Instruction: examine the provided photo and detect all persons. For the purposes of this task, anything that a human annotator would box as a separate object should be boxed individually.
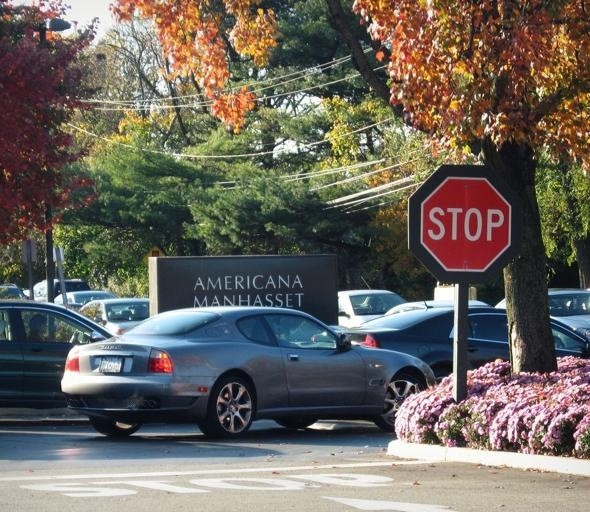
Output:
[26,313,48,342]
[369,297,385,313]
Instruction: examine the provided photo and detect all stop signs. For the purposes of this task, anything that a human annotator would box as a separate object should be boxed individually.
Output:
[419,176,512,273]
